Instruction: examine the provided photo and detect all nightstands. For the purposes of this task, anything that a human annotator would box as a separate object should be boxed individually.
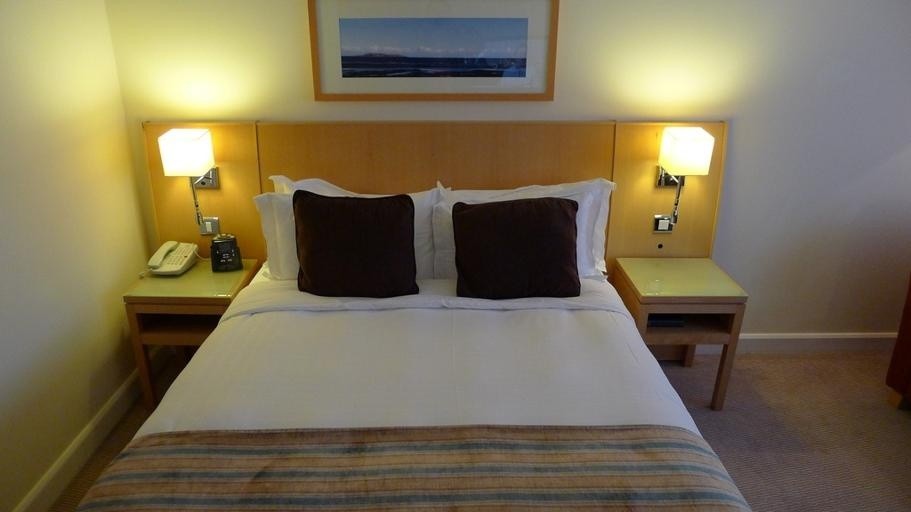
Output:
[123,258,261,411]
[613,259,749,412]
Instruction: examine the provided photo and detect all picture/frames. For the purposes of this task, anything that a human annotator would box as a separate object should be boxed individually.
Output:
[307,0,560,102]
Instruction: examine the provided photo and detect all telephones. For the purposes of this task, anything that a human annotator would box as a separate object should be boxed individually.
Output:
[147,241,198,275]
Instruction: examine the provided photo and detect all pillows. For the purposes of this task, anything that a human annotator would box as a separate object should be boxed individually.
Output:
[265,174,371,194]
[434,177,616,282]
[253,192,437,281]
[451,197,583,297]
[294,190,420,299]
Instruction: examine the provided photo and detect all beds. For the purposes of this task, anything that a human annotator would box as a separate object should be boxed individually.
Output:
[75,262,750,512]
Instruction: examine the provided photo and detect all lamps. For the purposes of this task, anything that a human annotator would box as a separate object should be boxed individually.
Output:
[656,126,716,226]
[156,128,220,226]
